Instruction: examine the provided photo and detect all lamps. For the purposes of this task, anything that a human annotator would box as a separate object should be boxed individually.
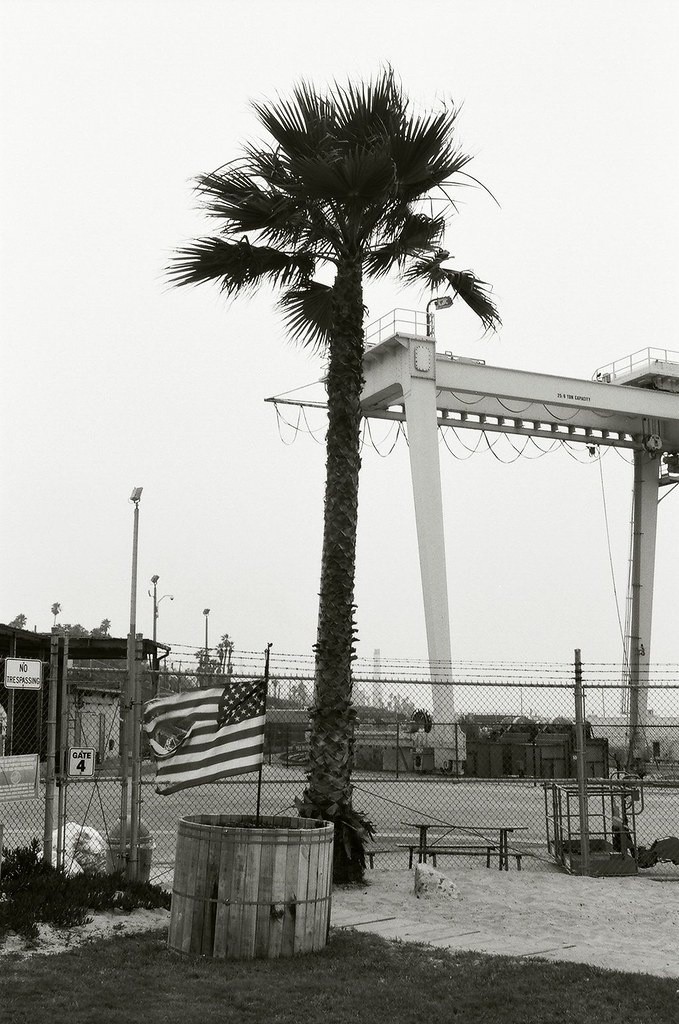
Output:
[427,296,454,337]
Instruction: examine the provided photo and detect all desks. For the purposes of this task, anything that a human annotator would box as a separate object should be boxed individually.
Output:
[400,821,529,871]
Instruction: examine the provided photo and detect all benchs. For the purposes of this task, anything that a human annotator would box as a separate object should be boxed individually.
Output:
[363,848,394,870]
[414,849,527,872]
[395,843,496,869]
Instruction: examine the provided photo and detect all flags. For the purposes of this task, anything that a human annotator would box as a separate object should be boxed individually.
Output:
[140,680,267,796]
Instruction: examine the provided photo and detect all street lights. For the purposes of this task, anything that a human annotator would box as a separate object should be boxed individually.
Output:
[201,609,210,685]
[125,486,146,883]
[152,593,174,674]
